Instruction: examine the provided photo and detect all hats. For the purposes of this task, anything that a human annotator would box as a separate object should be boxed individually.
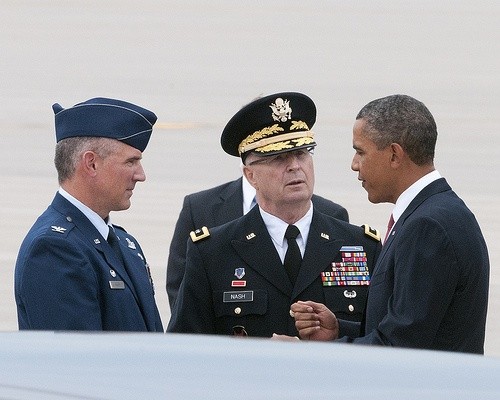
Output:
[221,92,316,167]
[52,98,157,153]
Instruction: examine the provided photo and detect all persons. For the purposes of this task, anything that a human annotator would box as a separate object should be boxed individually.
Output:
[14,97,165,333]
[271,94,490,355]
[165,90,384,342]
[166,175,349,314]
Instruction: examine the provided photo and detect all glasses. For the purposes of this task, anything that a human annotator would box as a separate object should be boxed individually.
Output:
[249,146,314,166]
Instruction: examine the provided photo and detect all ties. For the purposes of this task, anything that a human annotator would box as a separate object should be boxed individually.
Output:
[284,225,302,289]
[385,214,395,242]
[107,225,124,266]
